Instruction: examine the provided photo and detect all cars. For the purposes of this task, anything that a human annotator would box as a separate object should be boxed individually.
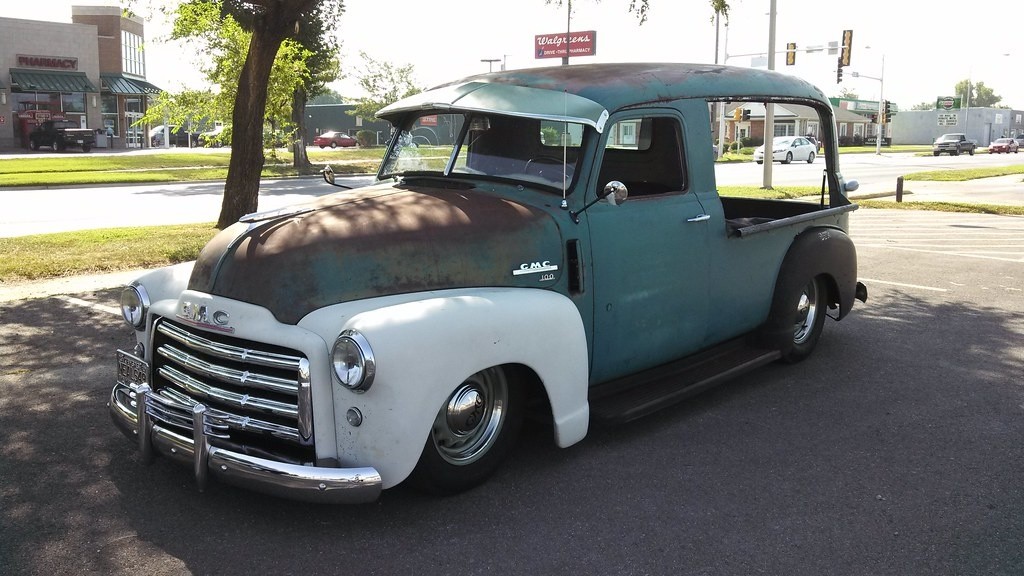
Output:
[313,132,358,149]
[1016,135,1024,146]
[126,128,144,142]
[987,137,1020,154]
[107,64,867,506]
[263,130,287,148]
[753,136,817,164]
[199,126,233,148]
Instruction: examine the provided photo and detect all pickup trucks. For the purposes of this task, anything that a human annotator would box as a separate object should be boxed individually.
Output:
[27,120,96,154]
[142,124,201,147]
[932,134,979,156]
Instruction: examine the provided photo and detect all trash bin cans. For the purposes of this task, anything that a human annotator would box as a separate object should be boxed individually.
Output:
[93,129,108,150]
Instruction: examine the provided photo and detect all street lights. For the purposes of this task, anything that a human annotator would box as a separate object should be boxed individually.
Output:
[481,59,501,72]
[964,52,1009,136]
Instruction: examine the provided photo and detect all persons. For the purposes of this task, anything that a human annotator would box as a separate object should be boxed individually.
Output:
[107,127,114,135]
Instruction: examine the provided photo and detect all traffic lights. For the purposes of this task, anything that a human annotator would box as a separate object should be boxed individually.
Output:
[884,101,891,124]
[837,59,844,83]
[743,110,751,122]
[734,108,741,121]
[873,115,878,123]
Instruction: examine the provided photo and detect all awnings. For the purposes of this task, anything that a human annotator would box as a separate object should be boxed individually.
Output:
[100,73,142,94]
[125,78,167,94]
[8,68,98,93]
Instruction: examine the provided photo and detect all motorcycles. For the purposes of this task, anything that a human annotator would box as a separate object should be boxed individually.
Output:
[386,131,419,149]
